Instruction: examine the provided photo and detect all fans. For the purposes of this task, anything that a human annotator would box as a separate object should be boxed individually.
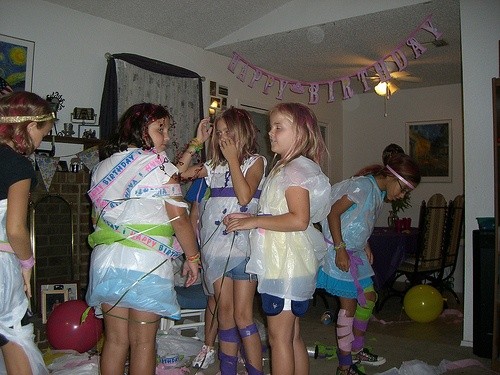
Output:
[352,69,421,84]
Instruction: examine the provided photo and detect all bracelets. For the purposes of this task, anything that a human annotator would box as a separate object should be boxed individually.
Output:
[187,136,203,153]
[333,241,346,252]
[184,250,201,263]
[18,256,36,269]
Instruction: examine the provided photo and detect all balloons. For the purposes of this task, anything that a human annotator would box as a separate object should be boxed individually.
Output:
[46,299,103,353]
[404,286,448,324]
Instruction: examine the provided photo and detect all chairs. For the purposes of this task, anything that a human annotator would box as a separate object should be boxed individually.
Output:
[380,194,447,316]
[159,284,206,340]
[426,194,465,304]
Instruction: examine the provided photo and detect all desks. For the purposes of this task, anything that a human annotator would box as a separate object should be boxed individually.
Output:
[367,226,419,313]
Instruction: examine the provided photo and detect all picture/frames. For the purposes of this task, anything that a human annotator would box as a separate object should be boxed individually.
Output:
[42,289,68,324]
[404,119,455,183]
[0,33,36,98]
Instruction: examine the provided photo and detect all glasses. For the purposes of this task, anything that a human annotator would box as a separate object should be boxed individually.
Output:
[397,179,406,193]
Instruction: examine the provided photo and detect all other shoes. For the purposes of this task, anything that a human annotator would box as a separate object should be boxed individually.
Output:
[336,364,366,375]
[352,348,377,362]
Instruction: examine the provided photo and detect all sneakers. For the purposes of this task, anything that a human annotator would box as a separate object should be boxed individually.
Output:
[192,344,216,369]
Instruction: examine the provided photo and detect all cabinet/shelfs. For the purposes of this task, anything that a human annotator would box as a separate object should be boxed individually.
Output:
[472,228,497,358]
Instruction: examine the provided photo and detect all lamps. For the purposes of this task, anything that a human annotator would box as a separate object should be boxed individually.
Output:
[373,81,402,97]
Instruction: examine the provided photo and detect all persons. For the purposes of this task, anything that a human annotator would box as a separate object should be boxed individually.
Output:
[86,104,200,375]
[223,102,331,375]
[0,91,58,375]
[174,108,268,375]
[314,153,422,375]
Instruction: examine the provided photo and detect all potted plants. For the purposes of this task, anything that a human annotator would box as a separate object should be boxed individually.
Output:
[387,191,411,229]
